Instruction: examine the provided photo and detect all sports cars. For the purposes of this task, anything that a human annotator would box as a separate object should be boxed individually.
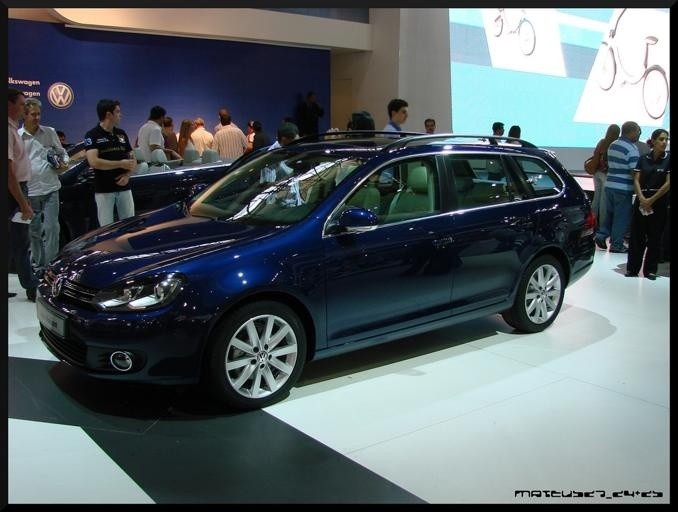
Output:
[7,139,233,290]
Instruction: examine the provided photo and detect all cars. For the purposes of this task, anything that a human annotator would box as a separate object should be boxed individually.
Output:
[29,130,595,408]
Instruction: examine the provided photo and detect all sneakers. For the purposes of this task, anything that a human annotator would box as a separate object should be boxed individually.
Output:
[593,234,608,250]
[609,243,629,254]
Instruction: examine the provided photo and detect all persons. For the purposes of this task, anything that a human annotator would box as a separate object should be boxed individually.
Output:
[8,88,302,303]
[380,99,409,181]
[350,111,375,138]
[591,121,670,280]
[506,125,520,145]
[297,91,325,137]
[425,118,435,134]
[485,123,504,181]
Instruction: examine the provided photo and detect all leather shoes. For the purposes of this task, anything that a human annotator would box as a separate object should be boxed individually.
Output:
[625,270,638,277]
[644,272,657,280]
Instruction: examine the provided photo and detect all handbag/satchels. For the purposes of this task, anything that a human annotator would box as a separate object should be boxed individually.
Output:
[584,154,600,176]
